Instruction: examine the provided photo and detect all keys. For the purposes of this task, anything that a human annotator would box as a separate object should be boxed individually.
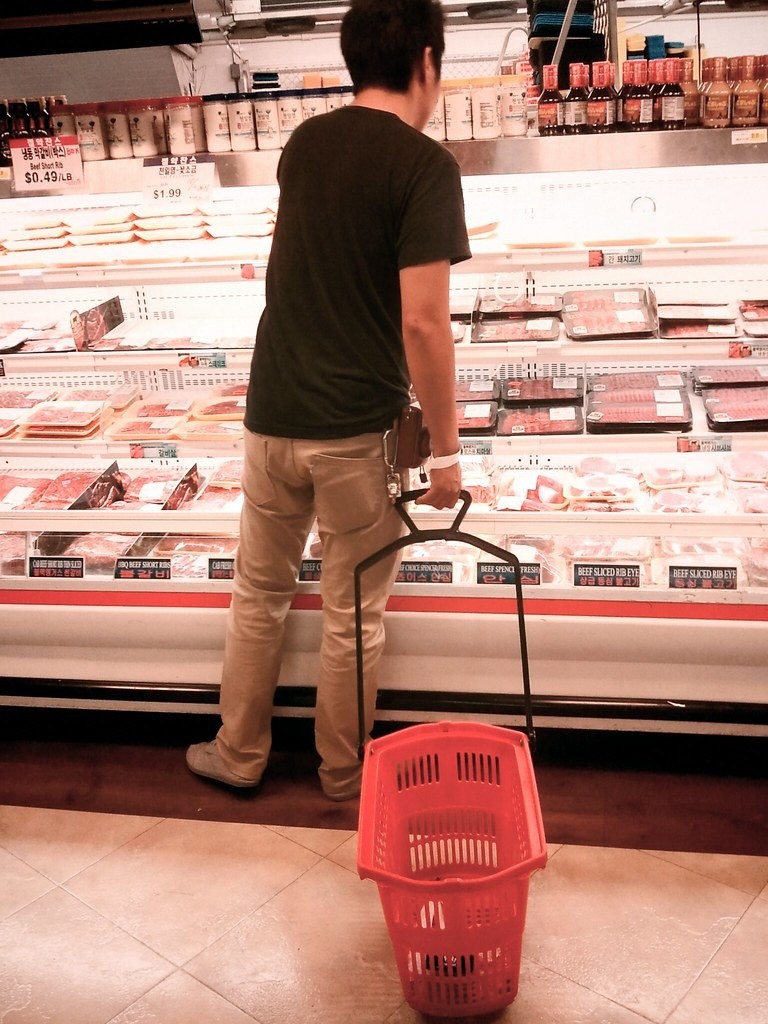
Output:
[385,474,401,505]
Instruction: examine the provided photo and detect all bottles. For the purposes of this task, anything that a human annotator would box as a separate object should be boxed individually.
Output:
[420,56,766,140]
[0,85,355,167]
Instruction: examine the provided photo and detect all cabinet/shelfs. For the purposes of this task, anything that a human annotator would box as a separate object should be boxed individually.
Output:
[0,30,767,731]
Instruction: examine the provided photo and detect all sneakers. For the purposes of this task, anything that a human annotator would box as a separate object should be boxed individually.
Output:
[185,741,262,797]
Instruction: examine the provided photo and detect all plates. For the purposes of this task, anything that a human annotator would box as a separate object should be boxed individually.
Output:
[1,192,280,253]
[0,284,767,588]
[466,212,767,247]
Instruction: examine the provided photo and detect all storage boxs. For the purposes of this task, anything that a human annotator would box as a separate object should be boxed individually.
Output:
[355,715,548,1024]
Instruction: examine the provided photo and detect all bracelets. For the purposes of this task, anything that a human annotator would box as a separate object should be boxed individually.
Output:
[429,443,464,470]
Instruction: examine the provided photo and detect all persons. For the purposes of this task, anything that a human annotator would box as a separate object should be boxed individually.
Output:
[187,0,474,802]
